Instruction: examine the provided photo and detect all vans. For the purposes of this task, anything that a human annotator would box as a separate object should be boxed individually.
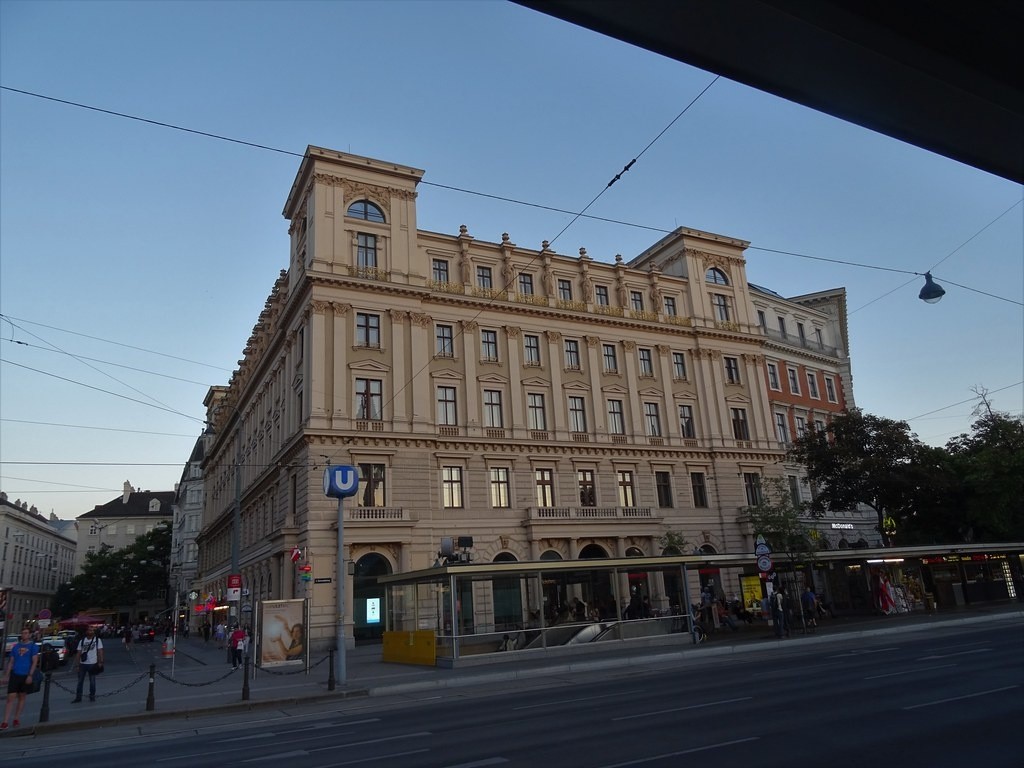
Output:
[58,630,76,640]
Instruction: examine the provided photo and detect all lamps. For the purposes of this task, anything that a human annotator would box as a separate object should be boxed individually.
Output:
[919,274,945,305]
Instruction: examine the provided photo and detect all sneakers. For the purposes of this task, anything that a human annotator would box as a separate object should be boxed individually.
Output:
[13,720,21,727]
[0,723,9,729]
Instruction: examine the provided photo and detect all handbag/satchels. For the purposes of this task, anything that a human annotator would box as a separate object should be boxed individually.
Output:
[23,680,41,694]
[91,663,105,674]
[121,637,126,643]
[80,652,87,662]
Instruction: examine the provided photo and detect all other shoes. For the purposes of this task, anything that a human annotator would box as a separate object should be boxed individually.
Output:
[239,664,243,670]
[90,697,95,702]
[72,697,82,703]
[231,666,238,670]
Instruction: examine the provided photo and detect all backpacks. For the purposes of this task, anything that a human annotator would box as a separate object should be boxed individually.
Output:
[770,592,779,611]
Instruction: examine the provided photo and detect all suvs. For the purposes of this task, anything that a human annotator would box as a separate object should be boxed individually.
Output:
[140,628,154,641]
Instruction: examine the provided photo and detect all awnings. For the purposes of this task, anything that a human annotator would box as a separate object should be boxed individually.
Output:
[159,606,175,616]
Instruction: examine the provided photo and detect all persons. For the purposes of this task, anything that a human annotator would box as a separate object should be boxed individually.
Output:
[770,586,794,639]
[499,628,526,651]
[599,595,617,622]
[574,597,586,622]
[695,587,726,626]
[71,628,104,703]
[214,620,252,669]
[458,253,664,309]
[0,628,40,729]
[525,611,549,637]
[198,621,211,646]
[147,621,190,639]
[802,586,819,627]
[272,614,304,660]
[552,605,560,625]
[97,624,143,650]
[621,583,655,620]
[749,592,761,611]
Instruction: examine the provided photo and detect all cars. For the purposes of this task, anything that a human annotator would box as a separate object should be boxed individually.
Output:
[5,637,20,653]
[43,640,69,665]
[35,643,59,672]
